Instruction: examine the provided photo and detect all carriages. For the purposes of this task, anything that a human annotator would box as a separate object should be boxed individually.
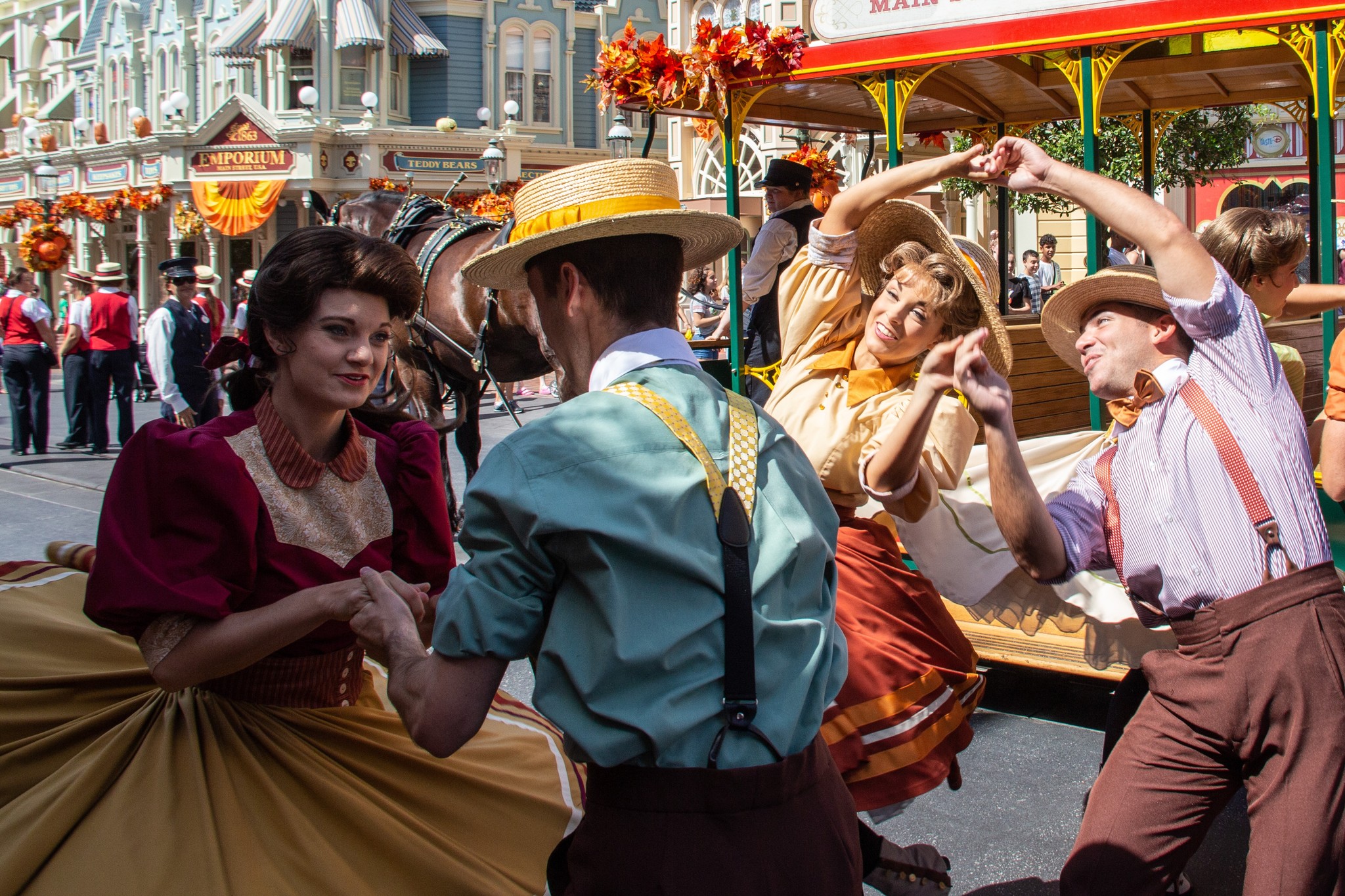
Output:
[309,0,1345,680]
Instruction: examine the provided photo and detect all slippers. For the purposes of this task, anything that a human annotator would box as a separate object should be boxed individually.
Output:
[516,387,534,396]
[538,386,552,394]
[443,405,454,411]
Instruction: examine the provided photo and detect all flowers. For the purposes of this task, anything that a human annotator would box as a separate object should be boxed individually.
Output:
[367,177,527,224]
[583,17,811,119]
[0,181,206,272]
[782,144,842,189]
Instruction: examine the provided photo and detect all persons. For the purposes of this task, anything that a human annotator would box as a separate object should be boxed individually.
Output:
[954,135,1345,896]
[441,383,453,410]
[0,267,59,456]
[368,341,395,410]
[516,375,551,396]
[232,269,259,368]
[493,382,524,414]
[762,143,1012,812]
[676,257,754,362]
[703,159,825,407]
[144,257,226,429]
[1196,207,1345,502]
[78,226,588,896]
[351,158,868,896]
[55,262,151,455]
[1108,229,1144,267]
[989,229,1066,315]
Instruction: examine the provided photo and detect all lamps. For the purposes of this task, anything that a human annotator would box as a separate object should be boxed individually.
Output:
[298,86,319,112]
[477,107,492,126]
[504,100,520,120]
[73,117,90,136]
[361,92,379,114]
[160,91,190,122]
[24,126,39,145]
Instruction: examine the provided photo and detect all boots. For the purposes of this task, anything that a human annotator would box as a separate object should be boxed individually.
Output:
[863,835,953,896]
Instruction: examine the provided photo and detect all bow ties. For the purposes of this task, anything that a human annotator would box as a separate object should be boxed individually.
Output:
[1104,368,1166,428]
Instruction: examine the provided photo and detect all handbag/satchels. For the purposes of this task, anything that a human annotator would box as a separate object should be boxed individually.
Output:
[1041,293,1055,304]
[684,328,693,340]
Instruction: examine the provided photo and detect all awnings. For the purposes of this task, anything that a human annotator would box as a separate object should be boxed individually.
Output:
[0,94,16,129]
[46,11,80,42]
[389,0,450,60]
[334,0,385,53]
[208,0,314,69]
[0,29,15,58]
[35,86,74,121]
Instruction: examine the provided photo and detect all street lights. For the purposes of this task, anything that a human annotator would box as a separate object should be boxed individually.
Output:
[34,154,60,342]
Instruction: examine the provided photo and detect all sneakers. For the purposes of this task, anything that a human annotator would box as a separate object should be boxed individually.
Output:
[56,441,94,449]
[494,399,524,414]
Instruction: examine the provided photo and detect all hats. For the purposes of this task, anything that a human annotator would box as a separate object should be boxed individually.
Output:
[92,262,129,281]
[59,290,67,296]
[1040,263,1172,376]
[60,267,98,285]
[194,265,222,288]
[236,269,258,288]
[752,158,814,195]
[857,198,1014,384]
[462,159,747,292]
[158,257,199,278]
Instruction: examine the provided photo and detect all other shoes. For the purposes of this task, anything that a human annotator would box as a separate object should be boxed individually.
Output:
[446,389,455,395]
[441,395,454,404]
[93,444,109,454]
[444,394,453,399]
[11,448,24,456]
[0,389,8,394]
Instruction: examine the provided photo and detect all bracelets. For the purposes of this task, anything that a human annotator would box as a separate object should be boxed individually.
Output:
[685,324,690,326]
[53,352,58,354]
[718,315,721,320]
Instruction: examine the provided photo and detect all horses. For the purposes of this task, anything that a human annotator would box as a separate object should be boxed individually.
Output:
[308,189,577,541]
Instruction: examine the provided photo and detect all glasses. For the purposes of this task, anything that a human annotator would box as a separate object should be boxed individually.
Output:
[167,275,196,286]
